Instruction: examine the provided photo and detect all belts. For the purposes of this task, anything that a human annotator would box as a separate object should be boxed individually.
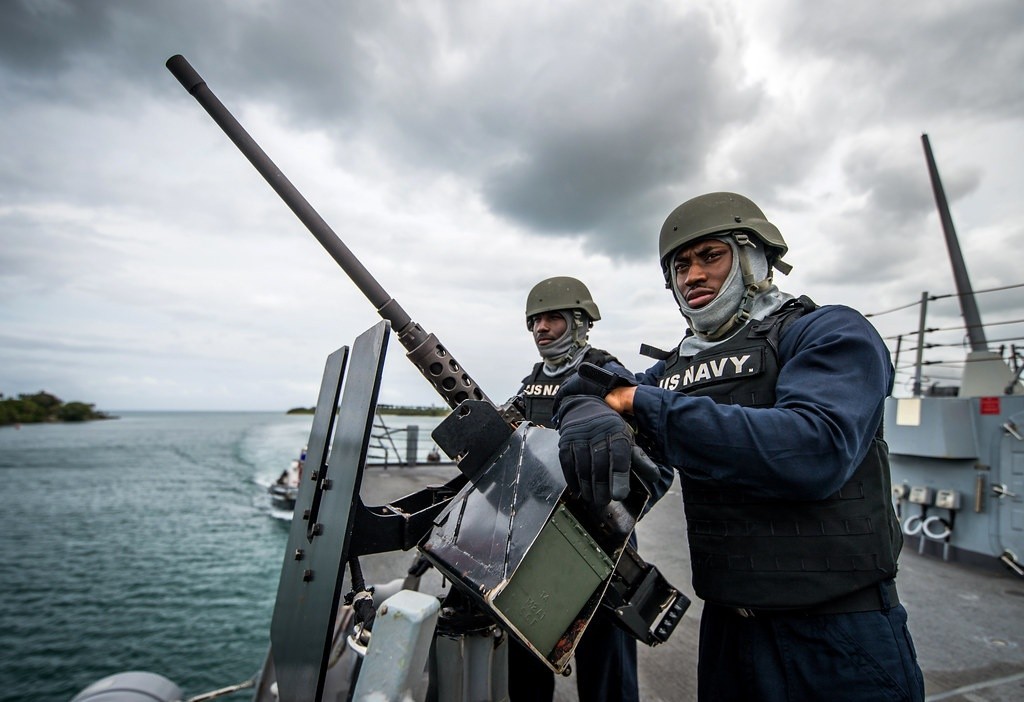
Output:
[732,583,900,620]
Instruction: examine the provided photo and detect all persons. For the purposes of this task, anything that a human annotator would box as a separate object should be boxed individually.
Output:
[507,277,674,702]
[551,191,927,702]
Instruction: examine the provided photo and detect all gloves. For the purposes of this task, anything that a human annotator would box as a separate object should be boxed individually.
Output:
[550,391,634,507]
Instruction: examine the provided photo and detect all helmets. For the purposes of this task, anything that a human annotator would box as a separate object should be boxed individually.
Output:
[659,191,788,261]
[525,276,601,320]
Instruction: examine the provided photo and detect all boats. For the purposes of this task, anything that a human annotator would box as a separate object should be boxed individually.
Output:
[269,461,299,512]
